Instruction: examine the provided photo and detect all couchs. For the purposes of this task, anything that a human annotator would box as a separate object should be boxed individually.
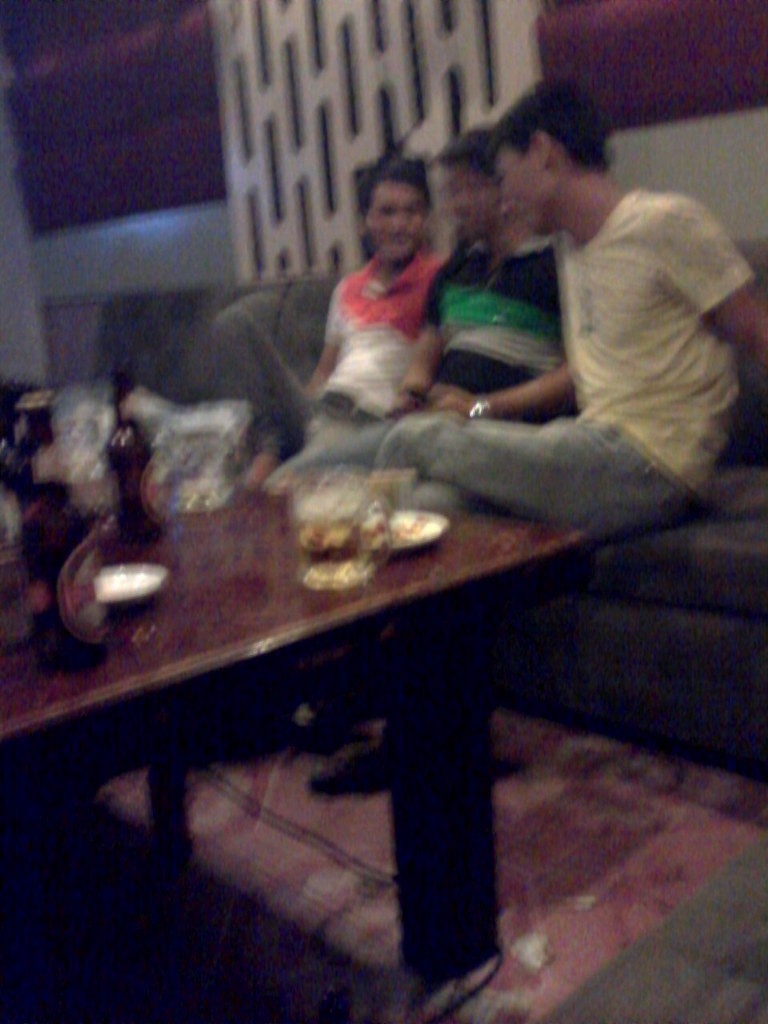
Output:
[198,236,768,783]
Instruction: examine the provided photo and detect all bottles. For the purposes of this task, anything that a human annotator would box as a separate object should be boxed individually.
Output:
[104,387,168,542]
[15,387,109,679]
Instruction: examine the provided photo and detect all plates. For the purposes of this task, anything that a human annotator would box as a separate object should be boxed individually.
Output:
[382,509,450,550]
[95,564,169,604]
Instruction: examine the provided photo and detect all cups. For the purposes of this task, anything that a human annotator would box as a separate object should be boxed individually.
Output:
[366,468,414,510]
[294,470,372,589]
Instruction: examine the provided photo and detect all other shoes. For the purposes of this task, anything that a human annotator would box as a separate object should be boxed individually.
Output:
[308,741,391,796]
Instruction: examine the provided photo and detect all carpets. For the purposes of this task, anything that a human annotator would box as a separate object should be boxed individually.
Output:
[95,703,768,1024]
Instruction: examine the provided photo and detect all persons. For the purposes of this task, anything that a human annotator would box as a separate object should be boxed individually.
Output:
[242,77,768,790]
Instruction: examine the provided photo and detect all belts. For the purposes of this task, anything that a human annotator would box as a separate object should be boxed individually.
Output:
[321,393,376,424]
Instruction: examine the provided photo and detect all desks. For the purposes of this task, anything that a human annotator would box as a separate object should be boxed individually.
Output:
[0,485,585,985]
